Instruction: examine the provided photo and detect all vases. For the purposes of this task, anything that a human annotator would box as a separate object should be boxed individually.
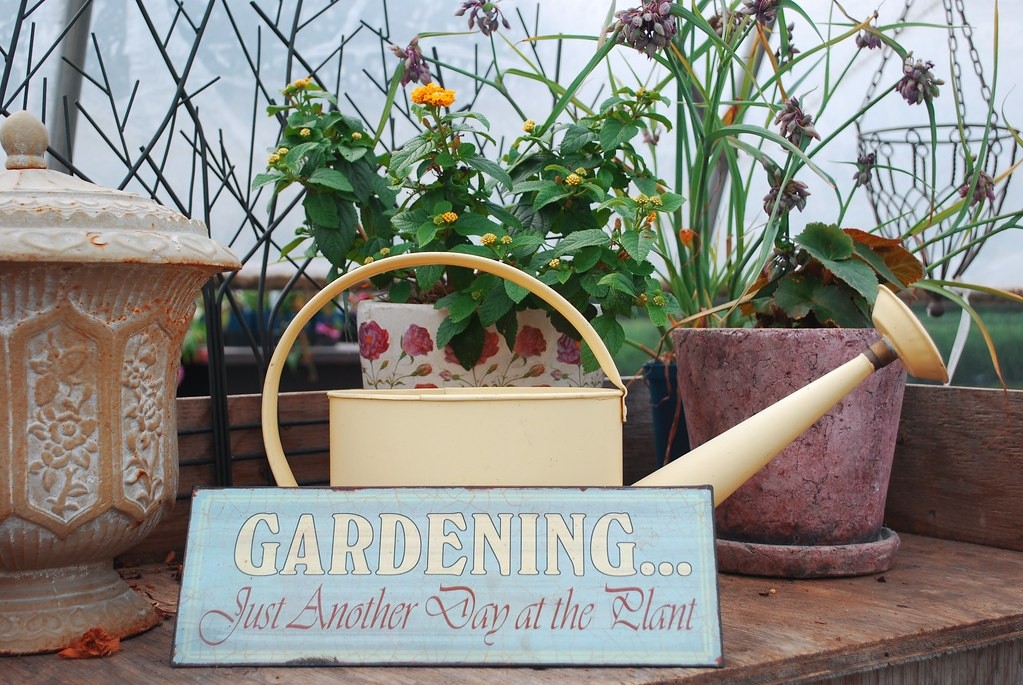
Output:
[668,323,911,576]
[354,294,611,389]
[327,384,626,487]
[643,359,690,468]
[241,0,1023,332]
